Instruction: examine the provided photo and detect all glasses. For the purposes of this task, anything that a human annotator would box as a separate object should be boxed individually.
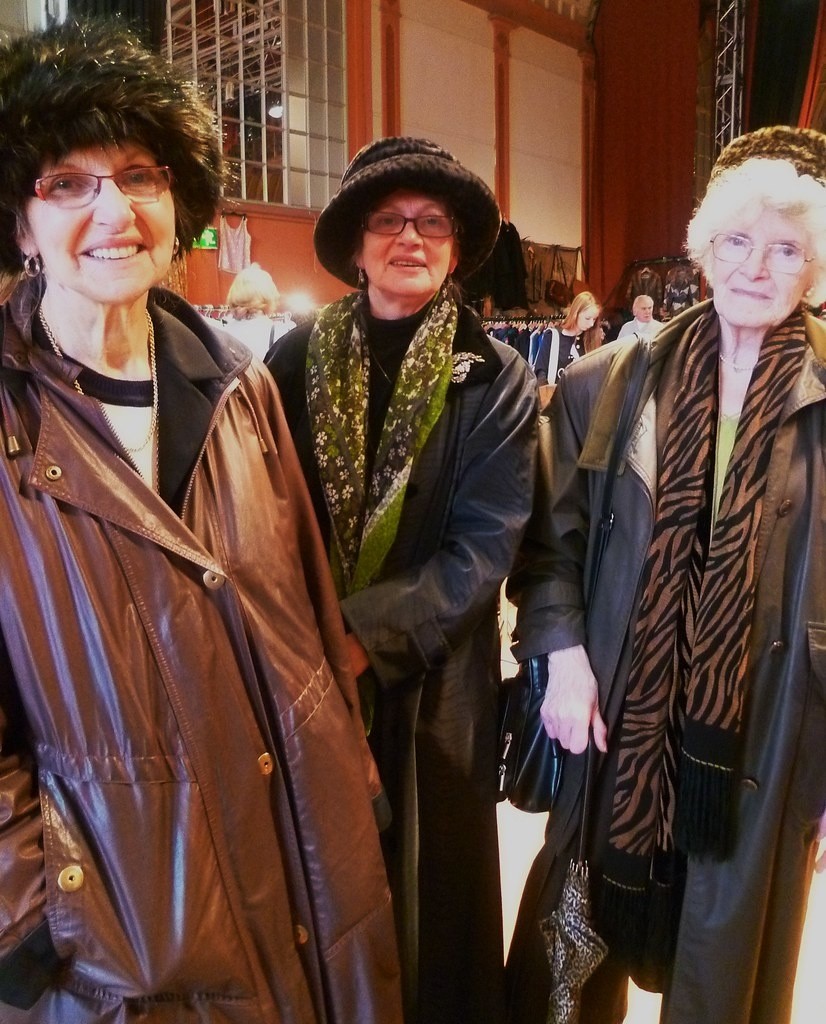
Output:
[361,210,464,239]
[29,165,174,210]
[709,232,816,274]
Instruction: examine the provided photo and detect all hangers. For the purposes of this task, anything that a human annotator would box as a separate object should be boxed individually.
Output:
[641,261,651,274]
[221,200,246,220]
[482,315,566,334]
[193,304,292,323]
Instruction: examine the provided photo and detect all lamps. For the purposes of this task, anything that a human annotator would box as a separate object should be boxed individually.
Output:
[225,51,234,102]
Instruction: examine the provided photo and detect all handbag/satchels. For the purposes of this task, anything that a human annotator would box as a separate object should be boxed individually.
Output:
[496,656,566,813]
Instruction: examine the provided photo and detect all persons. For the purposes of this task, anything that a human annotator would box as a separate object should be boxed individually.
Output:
[616,294,664,342]
[537,291,599,386]
[218,264,288,360]
[503,126,826,1024]
[261,138,543,1024]
[0,16,401,1024]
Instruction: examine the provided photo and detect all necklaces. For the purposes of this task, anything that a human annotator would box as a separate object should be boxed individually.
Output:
[37,305,157,449]
[369,350,393,386]
[719,357,755,373]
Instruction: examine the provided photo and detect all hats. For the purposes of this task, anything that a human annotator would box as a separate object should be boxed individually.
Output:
[0,13,245,271]
[314,136,503,289]
